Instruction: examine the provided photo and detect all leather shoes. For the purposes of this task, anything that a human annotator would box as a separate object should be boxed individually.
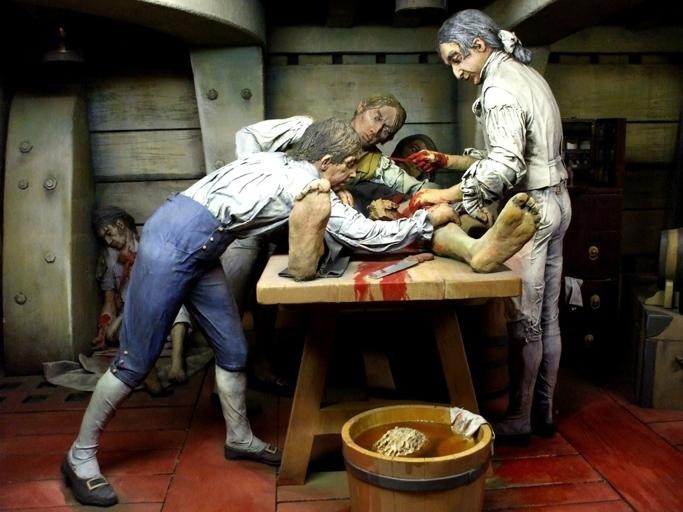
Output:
[60,454,119,506]
[224,444,282,465]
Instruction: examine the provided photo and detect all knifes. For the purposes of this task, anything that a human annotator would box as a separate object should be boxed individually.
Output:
[375,253,433,280]
[386,156,413,164]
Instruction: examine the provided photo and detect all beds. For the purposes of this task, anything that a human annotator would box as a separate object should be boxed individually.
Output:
[252,244,533,486]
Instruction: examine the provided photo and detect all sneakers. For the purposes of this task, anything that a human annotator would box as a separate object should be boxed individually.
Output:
[494,423,556,449]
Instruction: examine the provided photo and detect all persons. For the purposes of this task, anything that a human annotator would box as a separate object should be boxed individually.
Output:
[207,91,447,414]
[285,130,543,285]
[89,201,193,393]
[402,7,575,453]
[56,115,363,508]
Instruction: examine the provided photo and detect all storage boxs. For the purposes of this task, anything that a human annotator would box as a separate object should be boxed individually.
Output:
[637,301,682,411]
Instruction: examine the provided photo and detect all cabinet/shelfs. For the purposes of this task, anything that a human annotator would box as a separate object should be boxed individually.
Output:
[558,112,629,394]
